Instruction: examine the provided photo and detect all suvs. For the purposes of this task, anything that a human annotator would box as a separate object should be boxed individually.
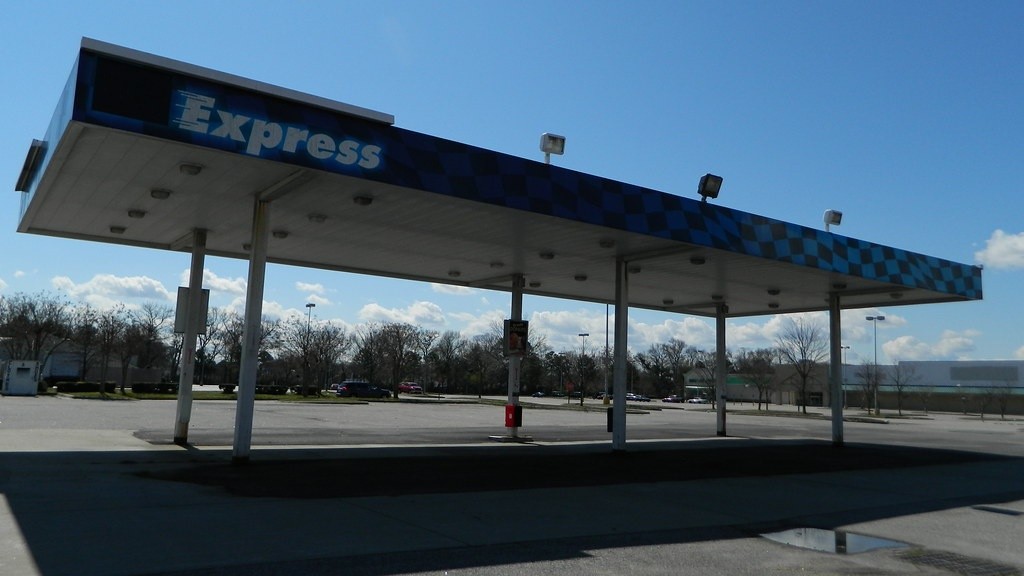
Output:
[336,380,392,399]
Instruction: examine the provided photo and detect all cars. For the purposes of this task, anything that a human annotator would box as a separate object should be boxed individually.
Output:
[597,391,614,400]
[399,380,424,394]
[662,395,683,403]
[330,384,339,389]
[626,392,651,402]
[552,390,564,397]
[572,391,582,400]
[687,397,709,405]
[532,391,545,397]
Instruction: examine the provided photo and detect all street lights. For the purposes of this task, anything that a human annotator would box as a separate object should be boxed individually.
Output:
[305,302,316,351]
[840,345,851,409]
[578,332,589,406]
[866,314,885,416]
